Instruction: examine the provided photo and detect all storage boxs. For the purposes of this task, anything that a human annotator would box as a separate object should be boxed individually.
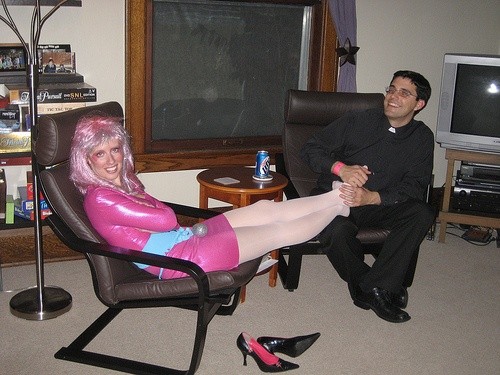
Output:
[9,81,97,104]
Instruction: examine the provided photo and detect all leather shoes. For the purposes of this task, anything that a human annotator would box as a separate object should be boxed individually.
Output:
[354,285,412,322]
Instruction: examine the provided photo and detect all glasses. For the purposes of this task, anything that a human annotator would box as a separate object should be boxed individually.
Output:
[384,86,418,99]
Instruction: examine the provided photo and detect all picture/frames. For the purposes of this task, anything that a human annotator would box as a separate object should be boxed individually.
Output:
[0,42,29,77]
[37,44,71,73]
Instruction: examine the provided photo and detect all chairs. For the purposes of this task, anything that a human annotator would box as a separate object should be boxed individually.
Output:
[276,89,435,292]
[35,101,262,375]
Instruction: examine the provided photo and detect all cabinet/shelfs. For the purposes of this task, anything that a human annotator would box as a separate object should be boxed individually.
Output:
[0,101,96,267]
[438,149,500,244]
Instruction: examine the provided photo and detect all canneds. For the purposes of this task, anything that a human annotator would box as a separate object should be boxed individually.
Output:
[256,150,270,178]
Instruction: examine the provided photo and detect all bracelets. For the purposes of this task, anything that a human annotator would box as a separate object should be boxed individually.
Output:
[334,162,345,176]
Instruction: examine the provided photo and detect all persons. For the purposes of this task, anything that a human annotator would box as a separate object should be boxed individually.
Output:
[303,70,435,323]
[69,116,368,279]
[0,54,22,69]
[44,59,72,73]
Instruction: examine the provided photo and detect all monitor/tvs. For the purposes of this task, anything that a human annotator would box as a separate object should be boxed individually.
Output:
[435,53,500,155]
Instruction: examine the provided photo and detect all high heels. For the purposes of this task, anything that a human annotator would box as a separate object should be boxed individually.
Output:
[236,332,300,373]
[257,332,321,358]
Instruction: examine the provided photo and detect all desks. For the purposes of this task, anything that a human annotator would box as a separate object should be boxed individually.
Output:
[196,167,289,301]
